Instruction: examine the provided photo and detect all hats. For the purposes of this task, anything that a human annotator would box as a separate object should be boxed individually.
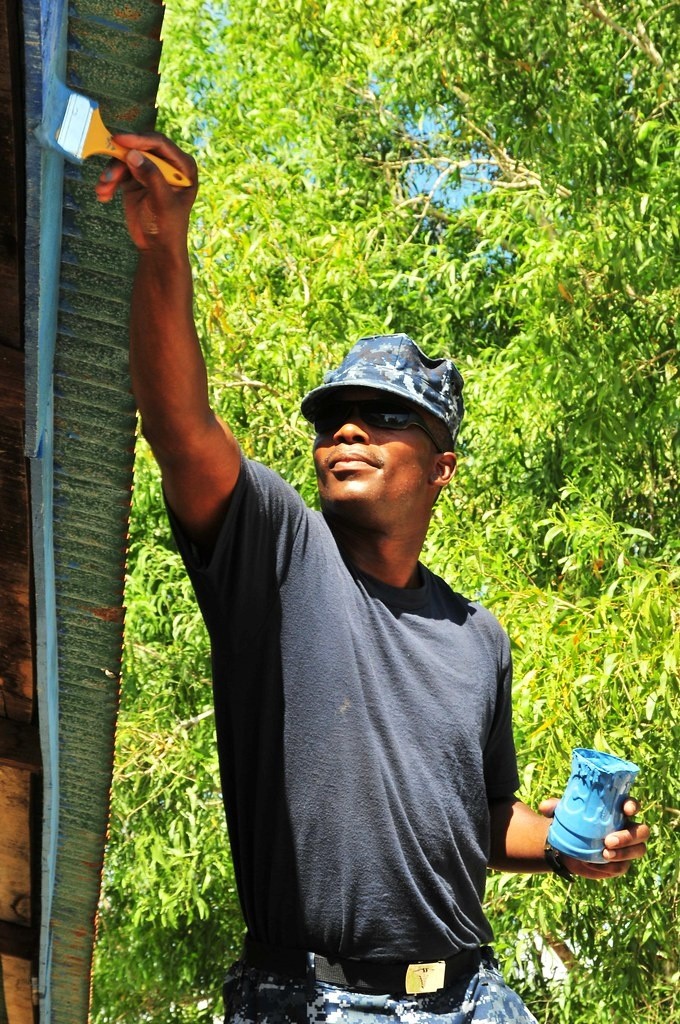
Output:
[302,332,463,443]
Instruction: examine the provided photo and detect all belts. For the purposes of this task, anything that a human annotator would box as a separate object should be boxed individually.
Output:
[243,933,480,997]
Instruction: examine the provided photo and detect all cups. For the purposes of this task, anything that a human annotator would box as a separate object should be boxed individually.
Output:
[546,747,639,865]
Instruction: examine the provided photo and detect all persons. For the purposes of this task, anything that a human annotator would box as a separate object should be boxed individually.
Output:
[98,127,650,1024]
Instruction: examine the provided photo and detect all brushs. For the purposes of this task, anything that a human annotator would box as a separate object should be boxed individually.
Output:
[33,71,192,187]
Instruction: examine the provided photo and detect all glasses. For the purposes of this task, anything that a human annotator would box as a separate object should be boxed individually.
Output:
[314,397,443,454]
